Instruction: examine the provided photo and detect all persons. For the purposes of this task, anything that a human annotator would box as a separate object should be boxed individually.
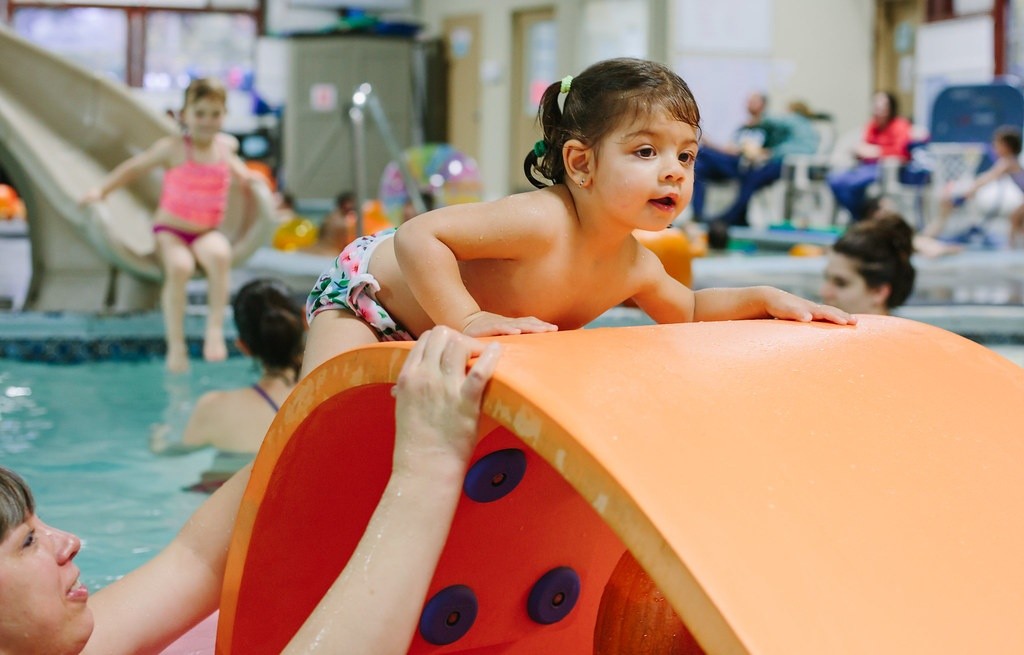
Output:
[301,58,858,386]
[83,83,1024,448]
[0,324,501,654]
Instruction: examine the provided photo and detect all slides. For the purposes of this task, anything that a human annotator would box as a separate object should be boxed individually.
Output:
[0,26,278,284]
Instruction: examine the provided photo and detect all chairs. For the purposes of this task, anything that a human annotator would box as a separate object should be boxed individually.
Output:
[746,115,837,224]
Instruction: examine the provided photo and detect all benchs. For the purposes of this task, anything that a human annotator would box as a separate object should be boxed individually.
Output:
[856,140,987,199]
